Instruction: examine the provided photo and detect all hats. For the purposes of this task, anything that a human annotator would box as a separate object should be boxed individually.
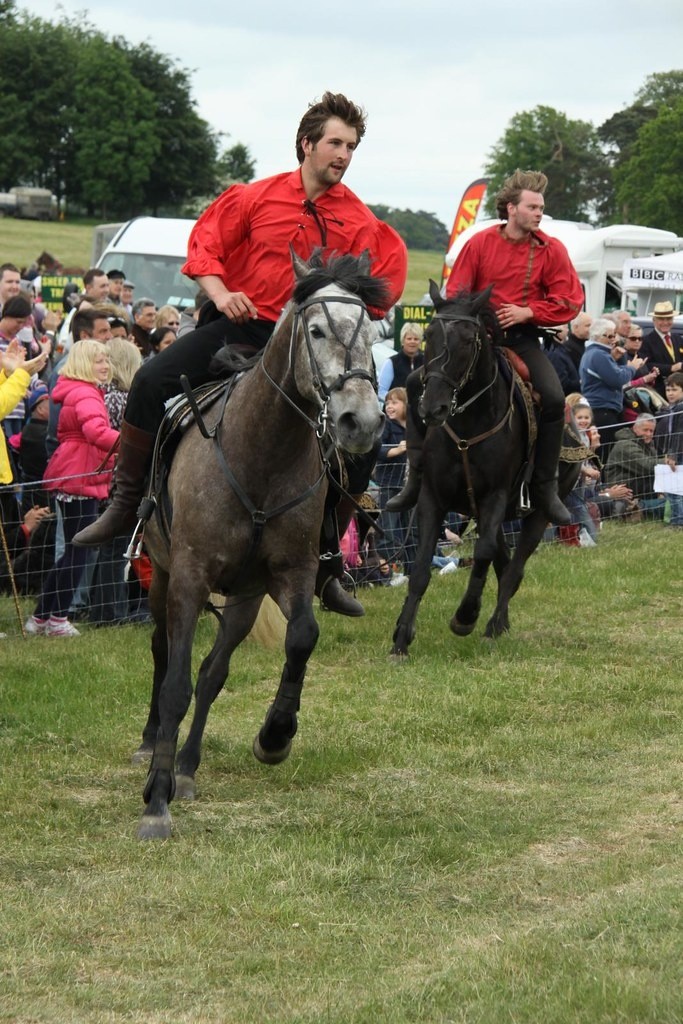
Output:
[123,279,135,289]
[648,301,679,318]
[28,386,50,410]
[107,270,125,280]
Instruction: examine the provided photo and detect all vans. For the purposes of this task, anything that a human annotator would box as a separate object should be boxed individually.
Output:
[46,215,203,369]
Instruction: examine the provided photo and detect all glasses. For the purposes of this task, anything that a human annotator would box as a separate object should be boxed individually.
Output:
[626,336,643,341]
[603,335,615,339]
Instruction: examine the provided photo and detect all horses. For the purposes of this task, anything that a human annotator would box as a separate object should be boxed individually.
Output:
[133,239,388,842]
[388,277,559,660]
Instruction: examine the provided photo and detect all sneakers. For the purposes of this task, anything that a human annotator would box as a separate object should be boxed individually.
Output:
[23,614,81,636]
[458,557,474,568]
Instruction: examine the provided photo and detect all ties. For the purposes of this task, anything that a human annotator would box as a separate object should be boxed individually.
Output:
[664,335,672,348]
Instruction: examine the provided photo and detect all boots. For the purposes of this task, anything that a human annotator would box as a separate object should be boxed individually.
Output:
[384,446,424,512]
[71,416,157,547]
[314,491,367,618]
[531,416,572,527]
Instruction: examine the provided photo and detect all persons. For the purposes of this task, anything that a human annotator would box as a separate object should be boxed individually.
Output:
[1,265,682,639]
[72,92,408,618]
[384,168,585,525]
